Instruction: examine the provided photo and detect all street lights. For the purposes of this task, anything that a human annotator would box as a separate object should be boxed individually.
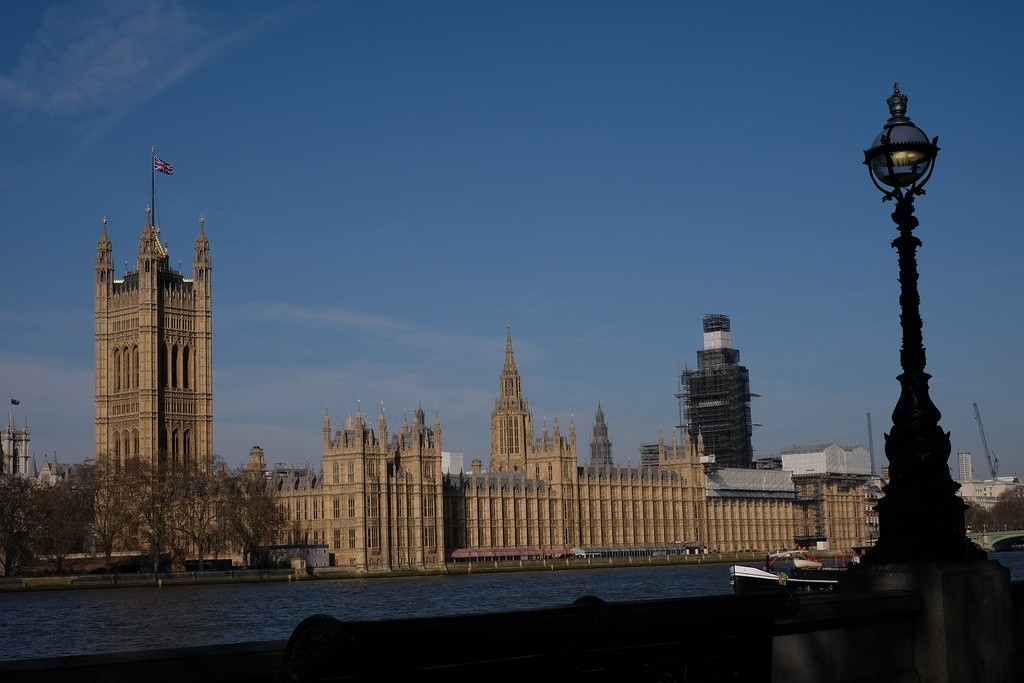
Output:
[856,85,991,567]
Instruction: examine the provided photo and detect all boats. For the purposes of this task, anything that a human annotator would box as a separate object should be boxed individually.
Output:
[727,548,851,592]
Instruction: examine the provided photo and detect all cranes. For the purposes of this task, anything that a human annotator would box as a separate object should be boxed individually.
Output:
[972,401,999,482]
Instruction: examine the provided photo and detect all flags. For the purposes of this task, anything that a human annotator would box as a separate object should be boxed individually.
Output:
[11,398,20,405]
[154,154,174,176]
